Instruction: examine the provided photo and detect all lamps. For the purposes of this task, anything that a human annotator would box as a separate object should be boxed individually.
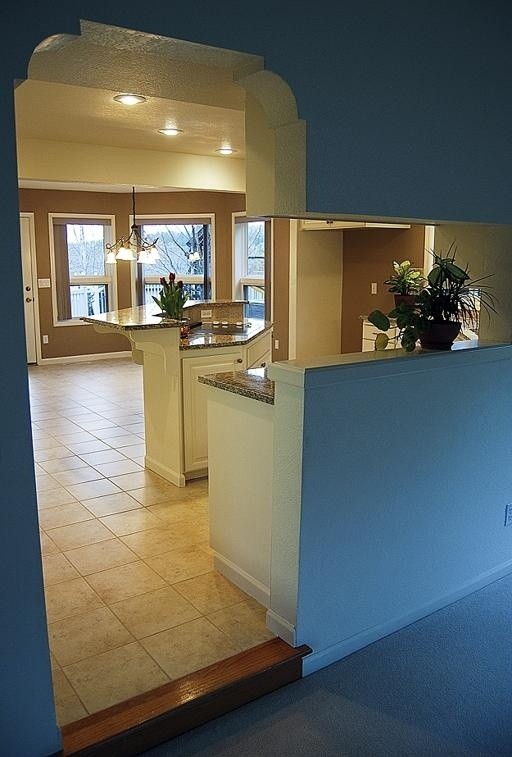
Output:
[105,187,161,265]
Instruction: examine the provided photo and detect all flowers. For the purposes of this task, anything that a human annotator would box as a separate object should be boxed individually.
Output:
[151,271,189,319]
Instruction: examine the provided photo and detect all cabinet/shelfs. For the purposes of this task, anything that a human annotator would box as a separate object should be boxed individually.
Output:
[203,382,275,611]
[180,323,276,478]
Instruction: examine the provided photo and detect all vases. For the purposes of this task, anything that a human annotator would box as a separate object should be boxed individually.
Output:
[180,322,189,343]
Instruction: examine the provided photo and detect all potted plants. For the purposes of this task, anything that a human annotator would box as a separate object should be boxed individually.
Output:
[370,235,500,352]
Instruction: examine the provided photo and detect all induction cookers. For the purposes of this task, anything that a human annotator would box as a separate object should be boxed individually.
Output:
[188,321,262,337]
